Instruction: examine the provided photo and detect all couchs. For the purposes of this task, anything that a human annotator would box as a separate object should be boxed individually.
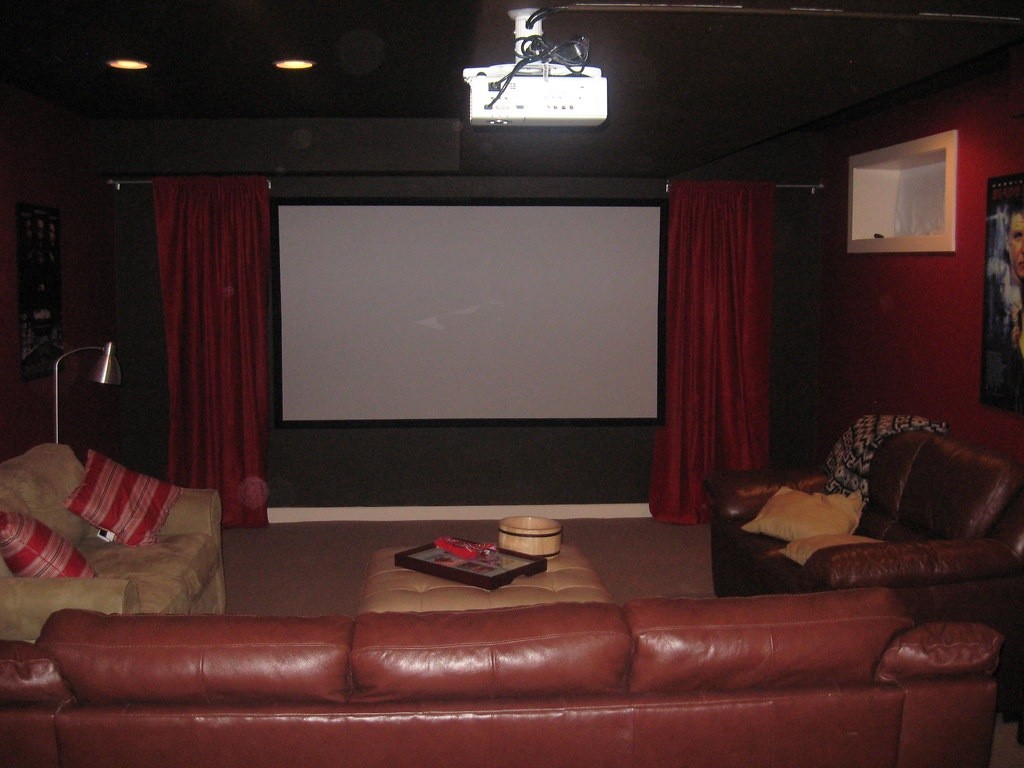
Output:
[704,415,1024,724]
[0,443,227,644]
[0,588,1005,768]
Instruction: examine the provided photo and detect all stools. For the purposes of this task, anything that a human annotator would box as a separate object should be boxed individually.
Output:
[360,538,616,613]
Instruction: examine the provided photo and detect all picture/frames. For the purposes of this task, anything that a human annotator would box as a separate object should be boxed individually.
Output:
[395,537,547,590]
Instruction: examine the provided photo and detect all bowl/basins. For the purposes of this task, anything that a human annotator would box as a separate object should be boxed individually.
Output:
[497,516,562,560]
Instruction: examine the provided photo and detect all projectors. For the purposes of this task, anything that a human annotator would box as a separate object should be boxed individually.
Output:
[469,76,607,127]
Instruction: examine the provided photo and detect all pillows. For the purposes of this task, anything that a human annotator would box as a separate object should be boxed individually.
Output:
[741,486,863,542]
[62,449,182,548]
[0,512,100,578]
[780,536,886,566]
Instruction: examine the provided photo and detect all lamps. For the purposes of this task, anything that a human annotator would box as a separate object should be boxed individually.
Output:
[56,342,122,444]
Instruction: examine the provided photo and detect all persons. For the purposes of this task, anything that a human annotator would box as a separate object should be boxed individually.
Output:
[24,216,58,321]
[986,209,1024,400]
